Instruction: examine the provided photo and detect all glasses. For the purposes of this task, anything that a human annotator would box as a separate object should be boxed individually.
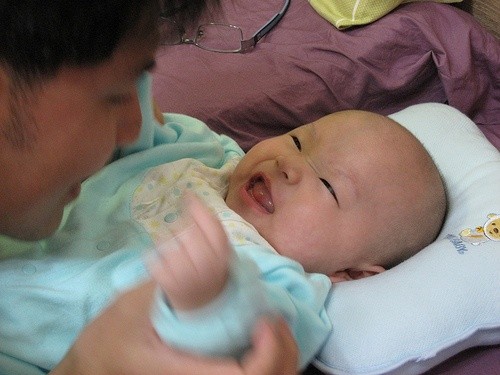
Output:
[158,1,290,54]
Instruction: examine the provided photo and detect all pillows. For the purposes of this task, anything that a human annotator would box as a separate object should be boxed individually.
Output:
[311,100,500,374]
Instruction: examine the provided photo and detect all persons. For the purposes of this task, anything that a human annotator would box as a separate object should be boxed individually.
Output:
[0,0,299,375]
[0,70,449,375]
